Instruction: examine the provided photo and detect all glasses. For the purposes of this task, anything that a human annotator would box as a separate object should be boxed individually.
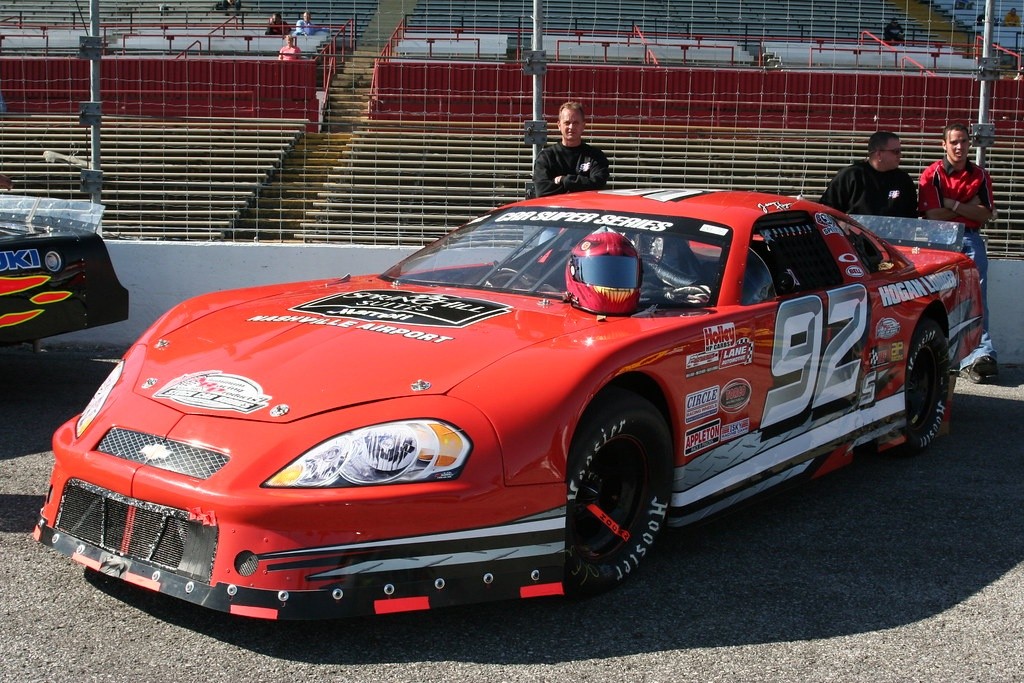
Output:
[872,148,902,154]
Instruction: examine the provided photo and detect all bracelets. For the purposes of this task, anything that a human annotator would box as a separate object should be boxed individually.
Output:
[952,200,960,212]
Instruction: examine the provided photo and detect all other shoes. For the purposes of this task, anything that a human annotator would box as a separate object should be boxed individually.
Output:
[964,354,1000,384]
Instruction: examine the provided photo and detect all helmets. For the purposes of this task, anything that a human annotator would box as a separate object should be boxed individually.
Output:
[565,226,646,317]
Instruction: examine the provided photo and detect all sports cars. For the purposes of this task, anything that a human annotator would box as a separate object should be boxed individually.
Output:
[32,186,999,623]
[0,193,129,346]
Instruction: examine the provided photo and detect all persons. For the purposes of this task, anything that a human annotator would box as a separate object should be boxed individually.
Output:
[278,35,303,62]
[818,129,917,219]
[885,0,1020,46]
[265,13,293,36]
[922,125,999,385]
[534,102,610,249]
[296,12,314,35]
[215,0,235,11]
